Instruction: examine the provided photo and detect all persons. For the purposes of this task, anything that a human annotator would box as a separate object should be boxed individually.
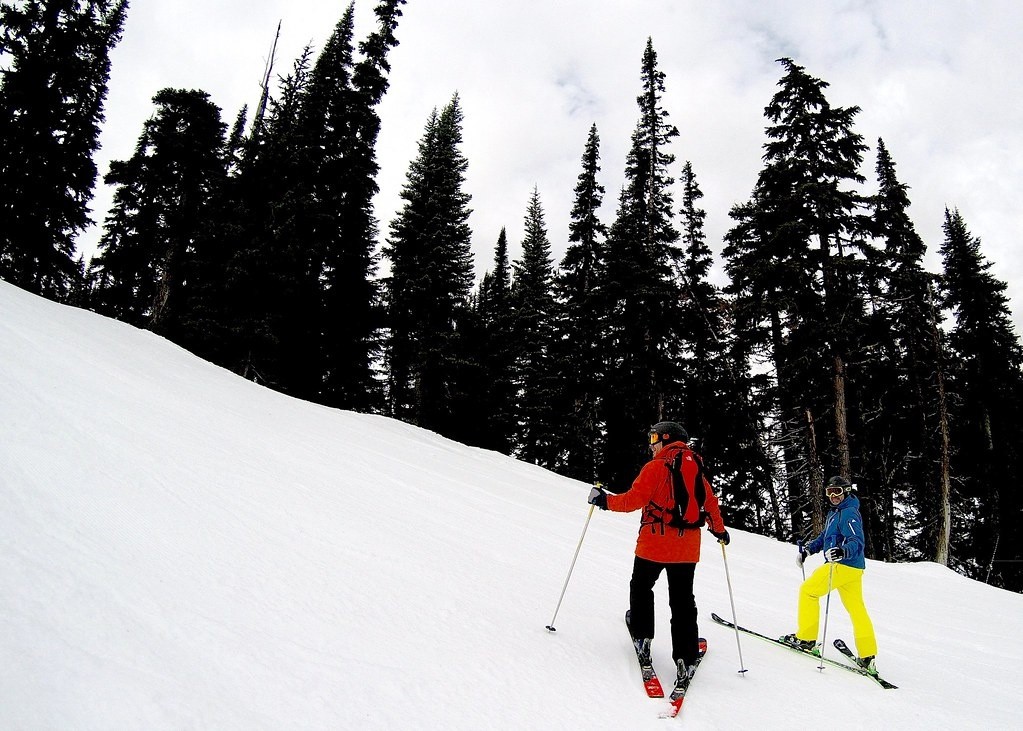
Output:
[588,421,730,667]
[784,475,878,670]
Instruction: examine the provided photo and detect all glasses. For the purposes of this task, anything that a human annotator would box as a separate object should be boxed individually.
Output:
[826,486,844,497]
[651,433,660,445]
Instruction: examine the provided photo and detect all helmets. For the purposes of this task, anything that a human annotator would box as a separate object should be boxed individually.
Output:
[828,477,850,499]
[650,421,688,448]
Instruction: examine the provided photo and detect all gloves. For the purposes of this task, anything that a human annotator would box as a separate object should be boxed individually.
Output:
[825,546,843,562]
[587,487,609,511]
[708,527,730,545]
[797,549,808,568]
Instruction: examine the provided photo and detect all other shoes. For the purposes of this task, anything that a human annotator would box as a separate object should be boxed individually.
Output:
[626,610,632,625]
[684,662,698,678]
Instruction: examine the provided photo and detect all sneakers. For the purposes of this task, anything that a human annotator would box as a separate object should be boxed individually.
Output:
[857,655,876,672]
[784,634,815,650]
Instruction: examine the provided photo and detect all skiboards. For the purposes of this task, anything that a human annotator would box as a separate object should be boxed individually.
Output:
[626,609,708,722]
[711,612,898,690]
